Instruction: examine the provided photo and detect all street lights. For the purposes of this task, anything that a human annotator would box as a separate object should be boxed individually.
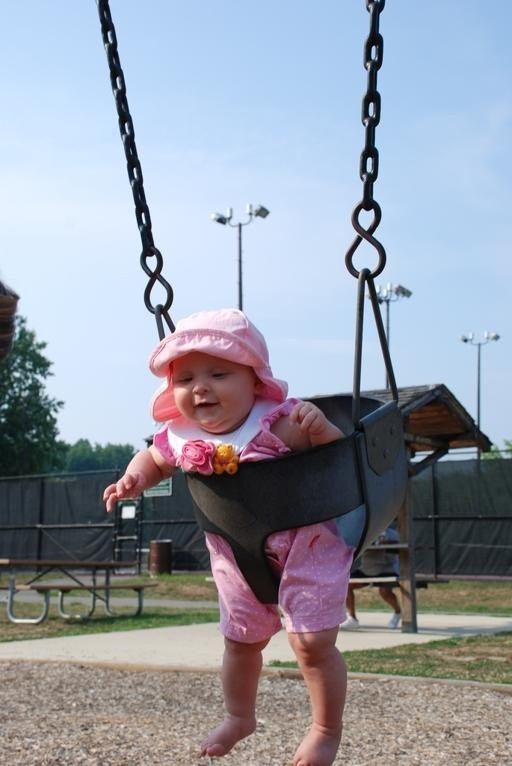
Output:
[370,282,415,395]
[209,200,270,316]
[458,328,502,460]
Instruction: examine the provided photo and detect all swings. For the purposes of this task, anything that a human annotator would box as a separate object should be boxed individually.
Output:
[94,0,406,603]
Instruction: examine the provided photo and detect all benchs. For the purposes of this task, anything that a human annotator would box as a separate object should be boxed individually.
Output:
[15,583,155,590]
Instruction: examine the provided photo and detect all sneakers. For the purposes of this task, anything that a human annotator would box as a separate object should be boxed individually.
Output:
[387,611,401,629]
[339,612,359,628]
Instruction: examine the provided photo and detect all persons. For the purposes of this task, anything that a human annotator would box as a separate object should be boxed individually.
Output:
[102,305,357,766]
[339,527,401,630]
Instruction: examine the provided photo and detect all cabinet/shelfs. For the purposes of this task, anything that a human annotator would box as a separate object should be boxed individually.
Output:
[350,543,409,583]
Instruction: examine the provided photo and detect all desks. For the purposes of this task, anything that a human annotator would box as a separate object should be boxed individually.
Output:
[2,558,142,624]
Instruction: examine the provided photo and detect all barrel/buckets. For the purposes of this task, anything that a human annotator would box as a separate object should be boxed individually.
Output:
[150,538,173,577]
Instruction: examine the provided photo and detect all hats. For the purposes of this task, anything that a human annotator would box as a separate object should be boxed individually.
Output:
[151,309,288,421]
